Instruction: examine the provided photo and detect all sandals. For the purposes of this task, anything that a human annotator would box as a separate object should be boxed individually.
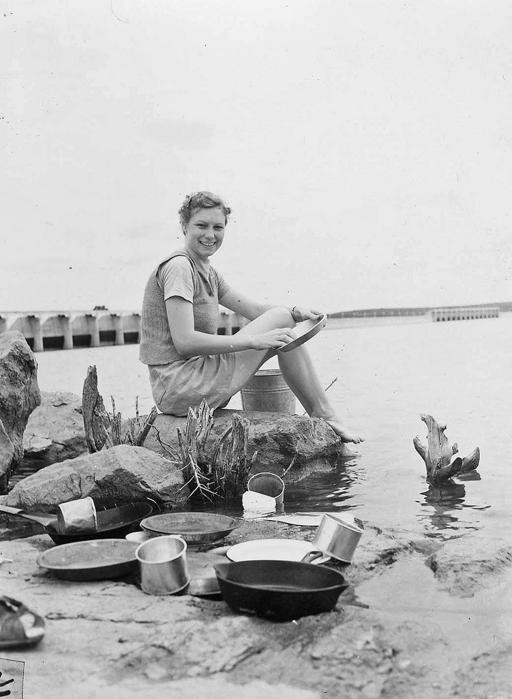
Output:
[0,591,49,653]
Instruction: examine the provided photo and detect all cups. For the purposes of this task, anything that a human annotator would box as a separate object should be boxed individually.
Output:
[312,513,365,563]
[57,496,98,534]
[136,534,191,596]
[247,471,285,505]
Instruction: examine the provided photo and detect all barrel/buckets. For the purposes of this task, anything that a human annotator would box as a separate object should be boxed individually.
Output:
[240,368,297,416]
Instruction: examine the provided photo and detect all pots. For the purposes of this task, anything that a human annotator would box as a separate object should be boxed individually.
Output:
[186,550,233,596]
[0,497,154,546]
[213,549,351,620]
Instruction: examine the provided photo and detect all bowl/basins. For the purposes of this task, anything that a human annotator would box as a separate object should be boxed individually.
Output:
[36,540,142,580]
[124,511,244,555]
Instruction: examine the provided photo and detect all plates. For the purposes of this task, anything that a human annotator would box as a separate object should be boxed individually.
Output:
[220,536,332,564]
[278,314,327,352]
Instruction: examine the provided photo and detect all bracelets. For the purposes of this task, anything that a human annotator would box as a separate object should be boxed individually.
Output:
[291,305,296,315]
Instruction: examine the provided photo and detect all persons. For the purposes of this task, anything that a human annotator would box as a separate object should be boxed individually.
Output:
[139,189,365,444]
